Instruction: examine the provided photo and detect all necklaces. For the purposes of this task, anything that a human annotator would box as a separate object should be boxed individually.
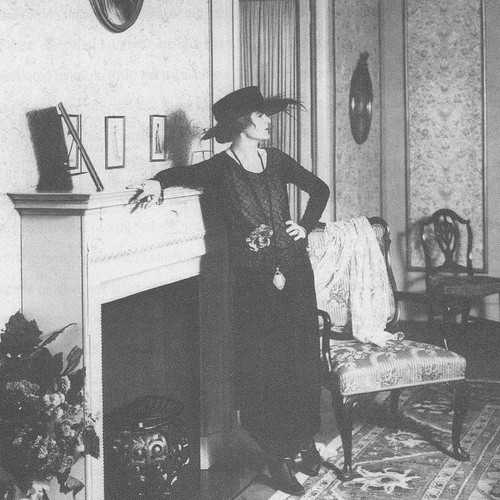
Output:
[230,144,285,290]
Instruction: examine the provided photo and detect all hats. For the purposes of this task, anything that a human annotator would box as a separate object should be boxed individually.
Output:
[199,87,305,140]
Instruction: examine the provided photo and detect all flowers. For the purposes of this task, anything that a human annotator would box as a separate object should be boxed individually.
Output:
[0,314,100,500]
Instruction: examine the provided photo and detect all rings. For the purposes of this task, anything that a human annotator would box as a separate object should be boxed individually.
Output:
[297,229,300,234]
[141,183,146,187]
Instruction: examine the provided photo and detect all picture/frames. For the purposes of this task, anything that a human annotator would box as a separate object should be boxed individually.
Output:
[60,114,82,175]
[106,115,125,169]
[150,114,167,162]
[88,0,143,33]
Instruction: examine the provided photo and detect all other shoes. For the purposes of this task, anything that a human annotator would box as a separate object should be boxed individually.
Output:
[300,438,321,476]
[270,458,305,496]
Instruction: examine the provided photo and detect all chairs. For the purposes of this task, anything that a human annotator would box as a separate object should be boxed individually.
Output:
[303,209,500,482]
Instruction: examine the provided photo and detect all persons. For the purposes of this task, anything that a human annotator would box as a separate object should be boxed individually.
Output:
[126,86,330,496]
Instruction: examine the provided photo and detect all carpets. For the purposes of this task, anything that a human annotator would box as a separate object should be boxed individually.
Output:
[268,372,500,500]
